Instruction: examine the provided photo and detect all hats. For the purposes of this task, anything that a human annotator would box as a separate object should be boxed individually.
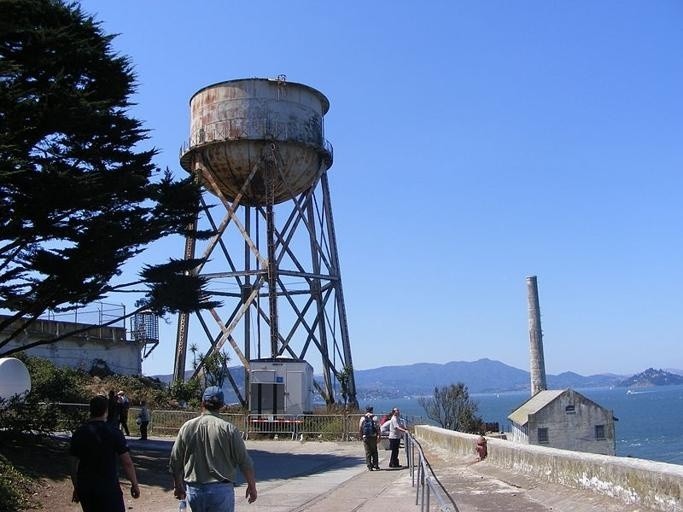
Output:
[202,385,225,407]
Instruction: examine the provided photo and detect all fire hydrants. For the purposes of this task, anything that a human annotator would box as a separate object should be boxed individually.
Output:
[474,433,487,460]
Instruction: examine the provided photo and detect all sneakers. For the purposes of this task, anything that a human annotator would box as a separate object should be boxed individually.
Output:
[367,462,403,472]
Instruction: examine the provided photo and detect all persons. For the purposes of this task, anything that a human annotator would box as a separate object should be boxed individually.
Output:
[105,390,119,431]
[357,405,382,471]
[168,385,257,512]
[387,406,406,467]
[117,391,130,437]
[66,394,140,511]
[137,398,149,440]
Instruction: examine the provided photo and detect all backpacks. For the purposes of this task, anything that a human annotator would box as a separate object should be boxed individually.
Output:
[361,415,377,443]
